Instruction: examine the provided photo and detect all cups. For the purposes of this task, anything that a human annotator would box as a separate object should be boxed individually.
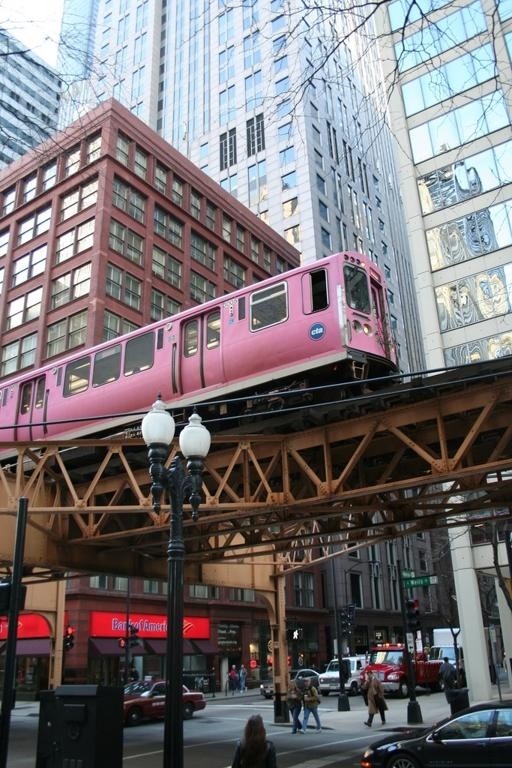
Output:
[304,695,308,701]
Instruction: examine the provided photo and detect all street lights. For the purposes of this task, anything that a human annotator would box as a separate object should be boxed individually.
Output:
[139,391,214,768]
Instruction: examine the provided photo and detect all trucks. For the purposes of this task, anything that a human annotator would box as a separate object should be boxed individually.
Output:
[361,642,446,696]
[428,628,466,683]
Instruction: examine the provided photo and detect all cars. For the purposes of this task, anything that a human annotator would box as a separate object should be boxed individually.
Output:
[122,677,206,727]
[260,667,321,698]
[361,701,512,768]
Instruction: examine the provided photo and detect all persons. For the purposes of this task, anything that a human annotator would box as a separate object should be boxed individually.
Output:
[438,657,458,696]
[237,664,249,694]
[228,664,240,696]
[284,678,305,735]
[229,714,277,767]
[117,666,126,684]
[128,666,140,682]
[363,670,388,728]
[300,674,322,735]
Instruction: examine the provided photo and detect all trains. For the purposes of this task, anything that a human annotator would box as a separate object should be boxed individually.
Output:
[0,250,403,472]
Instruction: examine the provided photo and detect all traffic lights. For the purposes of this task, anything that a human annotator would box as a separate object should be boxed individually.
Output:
[64,623,75,651]
[288,626,305,642]
[129,625,139,646]
[408,597,422,630]
[339,610,352,636]
[118,637,127,648]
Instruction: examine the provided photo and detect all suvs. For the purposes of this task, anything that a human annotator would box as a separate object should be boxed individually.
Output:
[319,654,372,695]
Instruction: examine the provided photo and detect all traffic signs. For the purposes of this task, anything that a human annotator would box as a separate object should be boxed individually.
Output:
[403,574,439,588]
[401,568,416,578]
